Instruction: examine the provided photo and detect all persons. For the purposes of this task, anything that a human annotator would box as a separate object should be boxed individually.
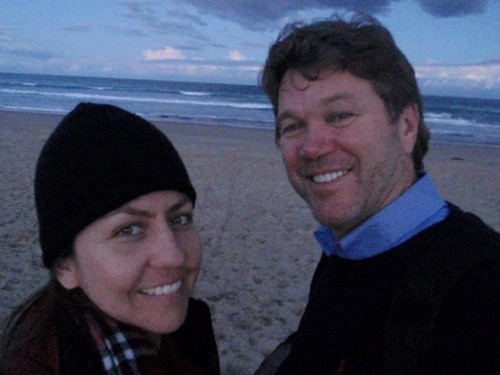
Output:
[254,9,500,375]
[0,101,221,375]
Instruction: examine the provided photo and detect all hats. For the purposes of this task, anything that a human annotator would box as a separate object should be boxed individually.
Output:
[35,101,197,267]
